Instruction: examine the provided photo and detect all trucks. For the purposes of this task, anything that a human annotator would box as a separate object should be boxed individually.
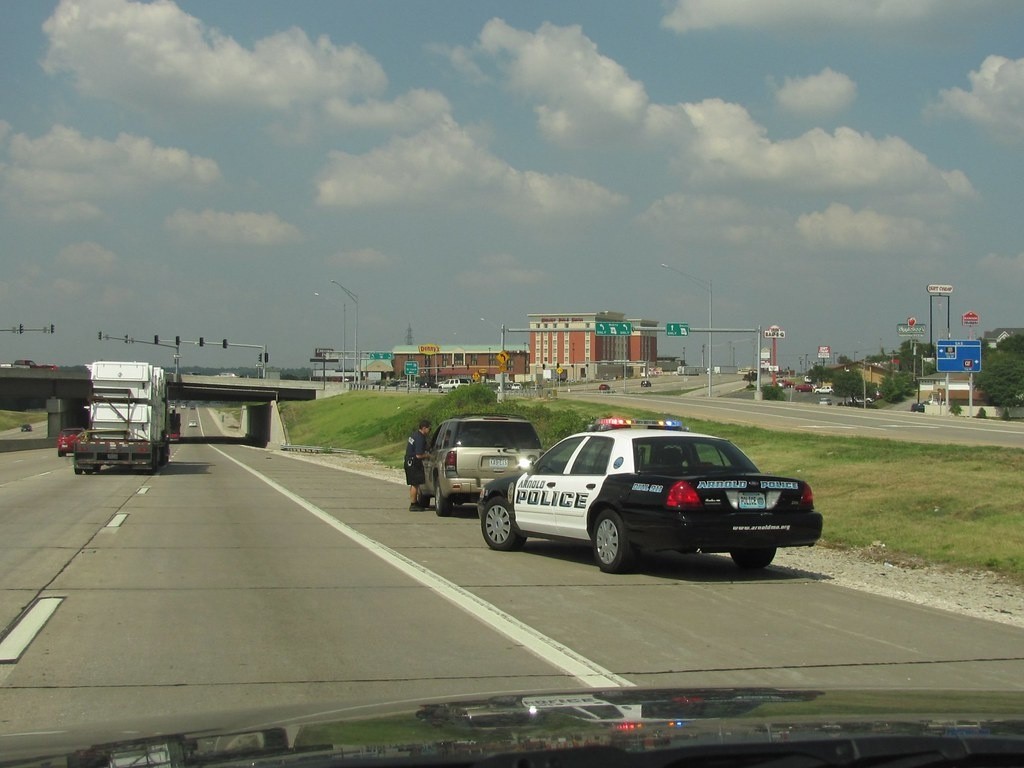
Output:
[169,412,182,441]
[71,362,171,475]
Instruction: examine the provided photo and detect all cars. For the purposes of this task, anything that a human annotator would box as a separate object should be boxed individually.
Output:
[1,357,57,370]
[511,382,523,390]
[170,404,176,410]
[190,405,195,410]
[599,384,610,390]
[180,404,186,409]
[477,415,824,574]
[530,383,544,390]
[21,423,32,433]
[641,380,652,388]
[911,403,927,413]
[213,371,241,378]
[765,375,883,409]
[188,420,197,427]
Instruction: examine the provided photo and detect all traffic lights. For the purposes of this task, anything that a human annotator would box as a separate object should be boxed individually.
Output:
[16,321,269,363]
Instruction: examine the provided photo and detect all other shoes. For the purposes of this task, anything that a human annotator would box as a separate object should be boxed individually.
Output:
[409,502,426,511]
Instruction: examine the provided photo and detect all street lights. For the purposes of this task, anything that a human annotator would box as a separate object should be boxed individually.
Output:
[659,262,714,398]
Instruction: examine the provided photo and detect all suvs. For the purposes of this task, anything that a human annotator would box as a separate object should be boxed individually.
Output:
[436,379,471,392]
[413,415,542,520]
[57,428,87,456]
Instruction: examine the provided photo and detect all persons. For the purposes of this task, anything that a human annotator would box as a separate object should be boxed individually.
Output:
[404,419,431,512]
